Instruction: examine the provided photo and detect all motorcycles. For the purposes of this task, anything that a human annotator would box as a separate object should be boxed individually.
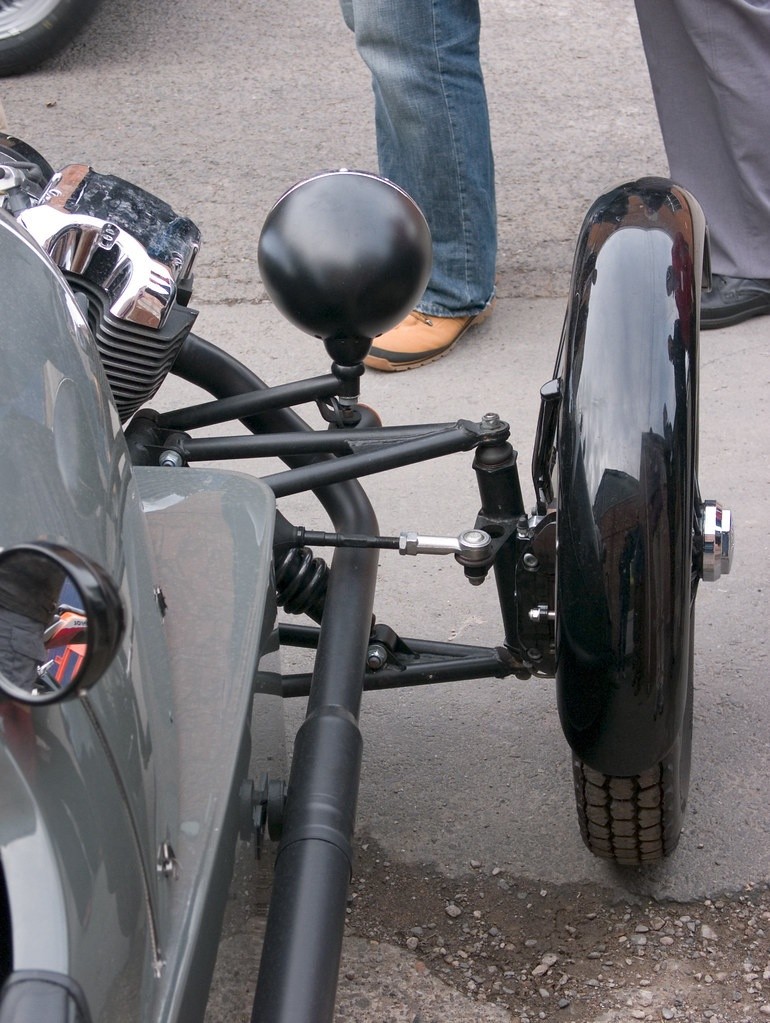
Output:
[1,132,735,1023]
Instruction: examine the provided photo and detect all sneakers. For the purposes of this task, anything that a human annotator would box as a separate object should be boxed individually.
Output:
[363,296,497,372]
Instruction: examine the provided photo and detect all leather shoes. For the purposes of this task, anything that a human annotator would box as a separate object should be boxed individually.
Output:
[699,274,770,331]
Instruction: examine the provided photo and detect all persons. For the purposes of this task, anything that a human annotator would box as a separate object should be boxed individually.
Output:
[339,0,495,374]
[635,0,769,330]
